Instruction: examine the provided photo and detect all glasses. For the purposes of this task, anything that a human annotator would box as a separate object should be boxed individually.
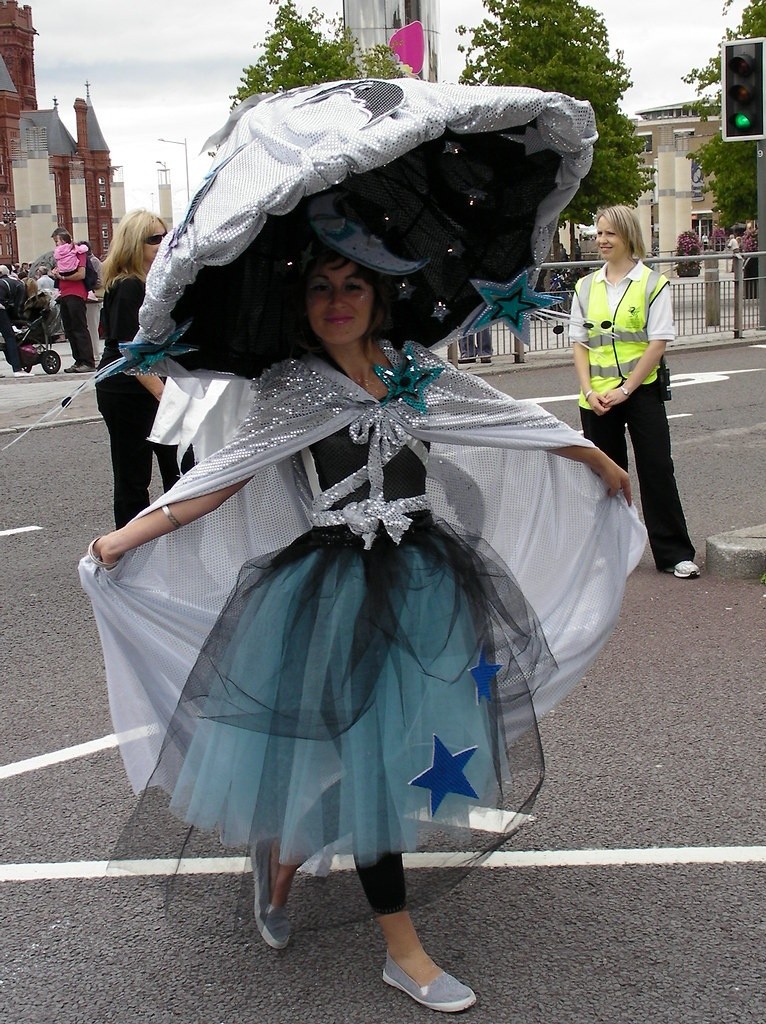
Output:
[144,232,167,245]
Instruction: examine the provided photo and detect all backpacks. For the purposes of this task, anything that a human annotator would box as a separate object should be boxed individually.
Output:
[78,241,97,291]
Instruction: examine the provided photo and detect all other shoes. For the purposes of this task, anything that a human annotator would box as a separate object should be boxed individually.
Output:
[14,370,35,377]
[87,290,98,302]
[51,288,61,301]
[251,837,290,949]
[0,374,5,378]
[383,948,477,1012]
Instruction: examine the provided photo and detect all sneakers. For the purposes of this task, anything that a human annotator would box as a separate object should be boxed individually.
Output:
[64,363,78,373]
[76,365,96,372]
[664,560,700,578]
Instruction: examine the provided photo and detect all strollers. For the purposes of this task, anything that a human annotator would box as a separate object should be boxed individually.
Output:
[0,288,64,374]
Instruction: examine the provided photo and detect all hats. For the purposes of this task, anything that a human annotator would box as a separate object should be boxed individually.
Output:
[308,162,431,276]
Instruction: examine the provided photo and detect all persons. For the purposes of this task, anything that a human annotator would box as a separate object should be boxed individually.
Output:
[559,243,568,261]
[571,206,702,579]
[50,228,96,373]
[53,233,88,276]
[80,78,648,1012]
[78,240,100,273]
[458,327,492,363]
[0,262,67,344]
[574,239,581,261]
[95,207,194,530]
[727,234,739,272]
[736,237,741,243]
[702,232,708,252]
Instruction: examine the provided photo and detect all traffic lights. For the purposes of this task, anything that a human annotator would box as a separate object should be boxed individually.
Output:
[719,38,764,139]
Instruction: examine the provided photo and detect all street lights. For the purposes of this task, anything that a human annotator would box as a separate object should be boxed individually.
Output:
[156,159,169,184]
[156,136,192,207]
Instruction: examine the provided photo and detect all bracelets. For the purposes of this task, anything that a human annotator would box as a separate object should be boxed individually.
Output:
[586,390,592,400]
[162,505,181,529]
[620,386,631,396]
